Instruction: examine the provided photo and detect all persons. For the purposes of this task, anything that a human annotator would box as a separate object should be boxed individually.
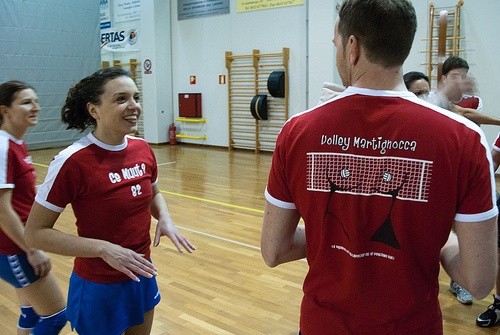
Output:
[24,67,197,335]
[261,0,499,335]
[403,57,500,328]
[0,80,68,335]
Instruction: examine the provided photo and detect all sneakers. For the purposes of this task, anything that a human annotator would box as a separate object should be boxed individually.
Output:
[449,280,474,304]
[477,295,500,327]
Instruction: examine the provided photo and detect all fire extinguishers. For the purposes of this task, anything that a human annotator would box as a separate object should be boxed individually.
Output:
[170,123,176,145]
